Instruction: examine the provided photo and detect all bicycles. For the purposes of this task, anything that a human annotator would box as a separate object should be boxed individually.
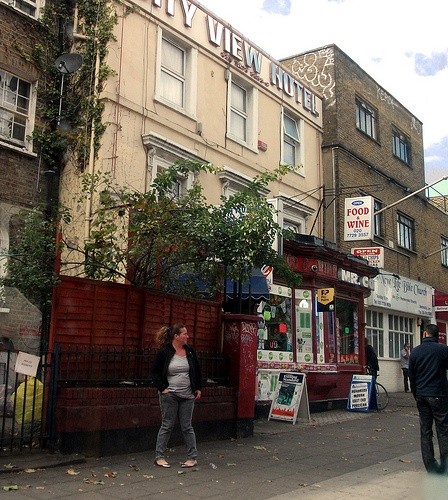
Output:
[375,374,389,410]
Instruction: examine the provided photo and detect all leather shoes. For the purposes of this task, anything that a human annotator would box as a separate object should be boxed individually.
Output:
[154,458,171,468]
[181,459,198,468]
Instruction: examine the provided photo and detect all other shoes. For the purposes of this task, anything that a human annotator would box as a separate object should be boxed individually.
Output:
[405,390,410,392]
[428,466,446,474]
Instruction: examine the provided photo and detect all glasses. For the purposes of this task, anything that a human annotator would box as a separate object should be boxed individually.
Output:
[405,346,411,347]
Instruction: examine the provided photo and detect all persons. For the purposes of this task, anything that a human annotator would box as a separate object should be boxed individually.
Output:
[400,344,413,393]
[408,323,448,474]
[153,324,202,468]
[365,338,379,379]
